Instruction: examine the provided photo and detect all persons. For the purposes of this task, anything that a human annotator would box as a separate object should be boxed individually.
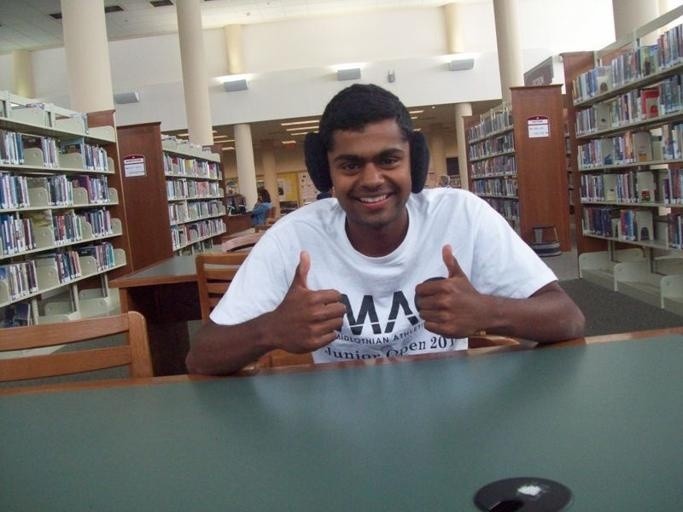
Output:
[185,84,586,378]
[226,186,246,212]
[250,187,273,226]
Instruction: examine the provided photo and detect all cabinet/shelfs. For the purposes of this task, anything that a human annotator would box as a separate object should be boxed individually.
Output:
[159,134,227,256]
[462,102,520,240]
[0,92,127,330]
[572,4,683,263]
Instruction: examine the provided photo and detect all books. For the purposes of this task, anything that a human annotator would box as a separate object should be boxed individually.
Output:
[577,161,682,206]
[575,122,683,166]
[464,107,520,139]
[573,75,682,135]
[53,211,82,245]
[170,200,223,226]
[165,178,220,199]
[481,197,519,219]
[172,219,224,251]
[1,129,25,164]
[36,251,82,282]
[24,136,59,169]
[158,153,227,179]
[0,303,31,327]
[0,260,40,297]
[570,23,683,100]
[78,174,110,205]
[47,174,74,206]
[61,139,109,172]
[0,172,30,209]
[84,210,113,239]
[469,154,516,176]
[78,241,115,273]
[0,213,34,255]
[470,174,518,197]
[468,131,514,159]
[579,197,682,249]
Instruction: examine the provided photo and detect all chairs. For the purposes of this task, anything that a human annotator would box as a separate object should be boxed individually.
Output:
[194,252,250,321]
[0,310,152,387]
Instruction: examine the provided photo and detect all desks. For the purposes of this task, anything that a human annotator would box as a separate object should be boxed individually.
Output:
[107,254,264,314]
[0,328,682,512]
